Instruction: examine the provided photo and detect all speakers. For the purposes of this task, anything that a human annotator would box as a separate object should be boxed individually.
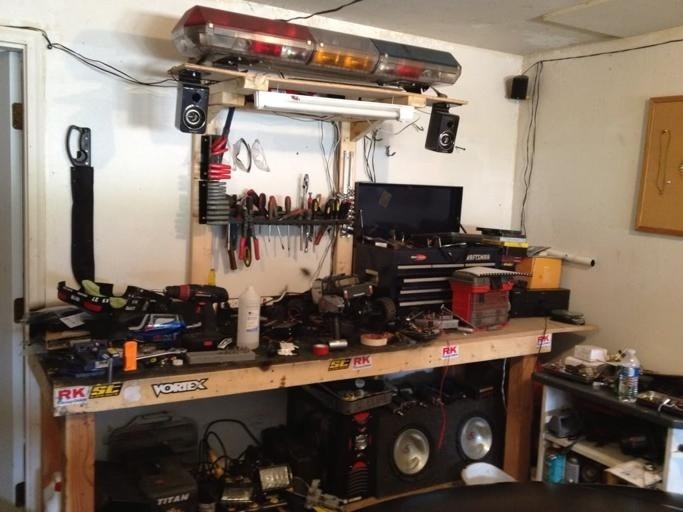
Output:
[286,388,506,500]
[425,112,461,154]
[175,85,210,135]
[511,76,529,100]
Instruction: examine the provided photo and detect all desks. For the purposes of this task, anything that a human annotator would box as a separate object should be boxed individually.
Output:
[28,317,597,512]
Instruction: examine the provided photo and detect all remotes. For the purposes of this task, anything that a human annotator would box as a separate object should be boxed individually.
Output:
[186,346,257,365]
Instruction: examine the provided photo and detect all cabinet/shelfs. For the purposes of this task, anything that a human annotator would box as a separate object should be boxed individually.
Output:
[533,372,683,493]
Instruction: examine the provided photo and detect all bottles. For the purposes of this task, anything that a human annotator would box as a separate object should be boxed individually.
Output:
[618,349,640,402]
[235,288,260,350]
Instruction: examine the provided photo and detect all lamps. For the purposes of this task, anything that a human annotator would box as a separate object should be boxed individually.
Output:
[254,90,415,122]
